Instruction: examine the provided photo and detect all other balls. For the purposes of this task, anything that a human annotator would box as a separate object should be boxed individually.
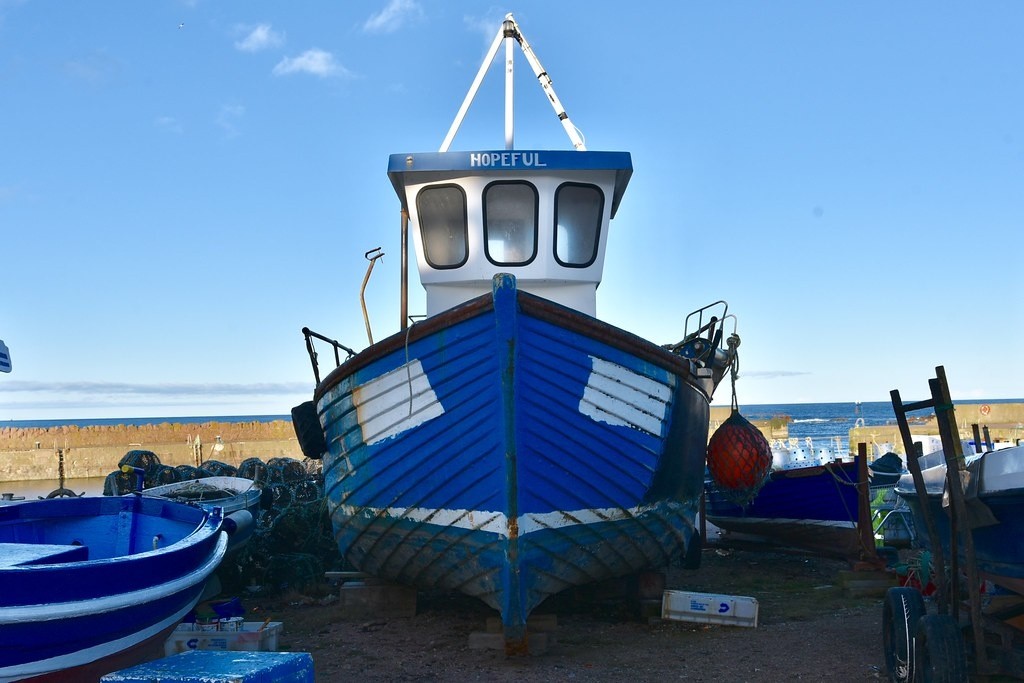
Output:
[706,420,773,491]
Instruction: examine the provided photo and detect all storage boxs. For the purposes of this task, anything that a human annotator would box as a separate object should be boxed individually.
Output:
[662,589,759,629]
[164,622,285,656]
[97,648,314,683]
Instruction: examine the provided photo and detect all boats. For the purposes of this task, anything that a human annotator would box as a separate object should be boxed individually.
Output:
[893,436,1024,594]
[702,441,878,568]
[108,475,263,568]
[0,463,228,683]
[290,12,739,653]
